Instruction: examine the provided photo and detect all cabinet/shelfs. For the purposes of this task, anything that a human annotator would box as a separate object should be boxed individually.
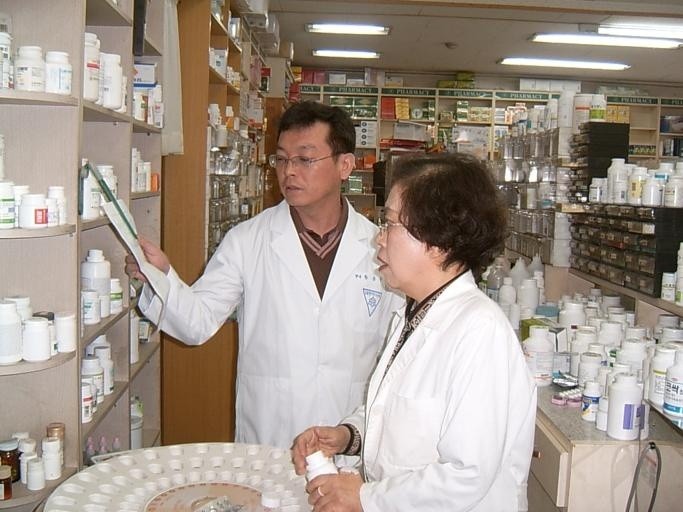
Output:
[525,380,681,511]
[159,1,274,448]
[293,81,683,213]
[0,0,166,512]
[482,89,682,295]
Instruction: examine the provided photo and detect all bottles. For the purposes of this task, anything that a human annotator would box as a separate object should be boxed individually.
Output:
[301,450,338,485]
[131,148,159,196]
[483,237,682,442]
[81,250,125,337]
[84,435,124,469]
[129,393,144,449]
[0,420,68,501]
[130,308,141,366]
[586,157,683,207]
[81,161,118,221]
[525,188,536,210]
[82,325,117,424]
[509,93,605,138]
[2,295,77,363]
[85,31,129,116]
[134,82,164,130]
[1,131,68,229]
[1,23,74,97]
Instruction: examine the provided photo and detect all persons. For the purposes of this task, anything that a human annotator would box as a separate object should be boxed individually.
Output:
[123,99,407,481]
[289,154,538,512]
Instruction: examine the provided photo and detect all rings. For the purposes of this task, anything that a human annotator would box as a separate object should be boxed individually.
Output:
[317,487,324,496]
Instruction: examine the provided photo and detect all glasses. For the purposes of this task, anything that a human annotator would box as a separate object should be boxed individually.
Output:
[269,154,334,169]
[377,216,409,236]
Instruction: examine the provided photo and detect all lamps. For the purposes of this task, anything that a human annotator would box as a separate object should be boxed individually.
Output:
[577,21,682,39]
[497,57,631,74]
[526,29,682,52]
[311,47,379,61]
[303,23,390,37]
